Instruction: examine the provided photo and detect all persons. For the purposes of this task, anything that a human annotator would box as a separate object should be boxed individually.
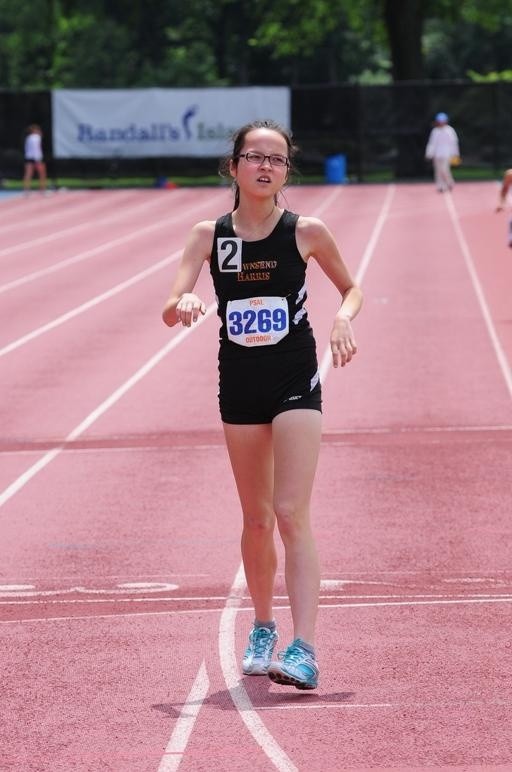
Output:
[425,111,461,194]
[494,167,512,249]
[162,120,366,692]
[20,123,48,202]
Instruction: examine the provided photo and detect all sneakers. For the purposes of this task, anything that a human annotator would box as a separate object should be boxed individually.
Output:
[267,639,320,689]
[241,625,278,676]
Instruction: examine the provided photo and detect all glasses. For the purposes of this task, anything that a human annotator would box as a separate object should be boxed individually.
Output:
[239,150,290,168]
